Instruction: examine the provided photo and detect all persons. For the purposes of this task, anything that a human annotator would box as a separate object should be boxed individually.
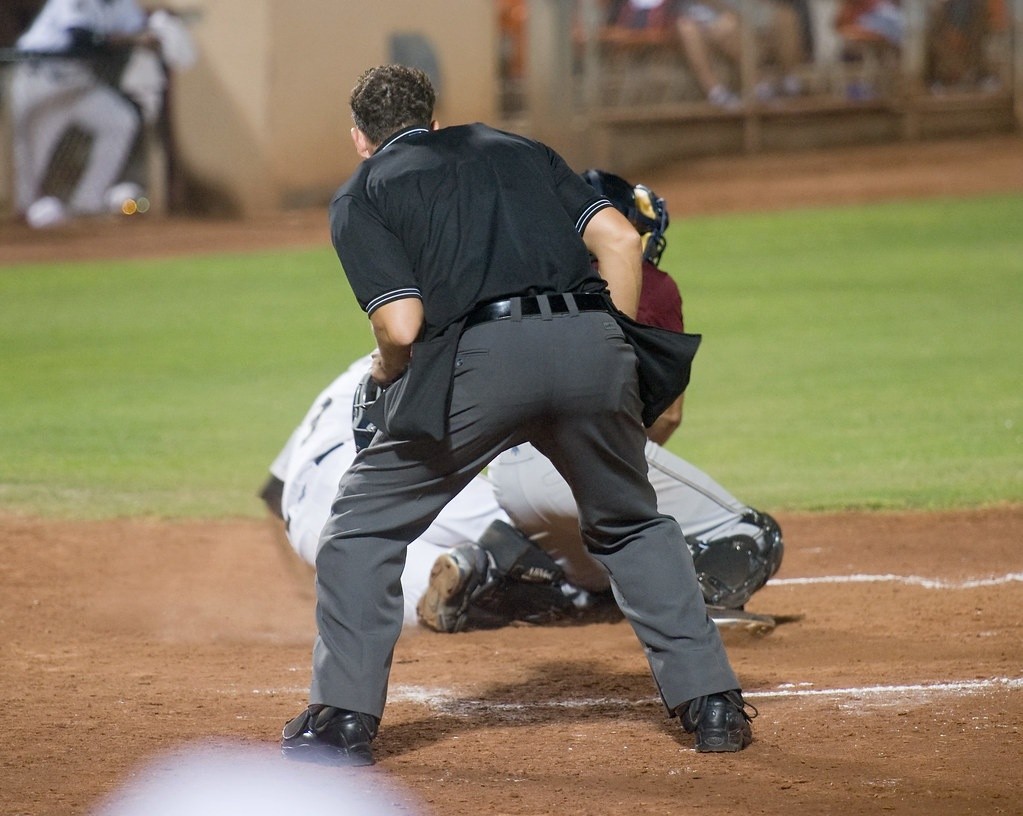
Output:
[8,0,160,215]
[601,0,996,95]
[256,167,786,644]
[276,63,761,767]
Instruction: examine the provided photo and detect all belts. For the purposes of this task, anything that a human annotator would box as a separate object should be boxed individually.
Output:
[468,291,608,321]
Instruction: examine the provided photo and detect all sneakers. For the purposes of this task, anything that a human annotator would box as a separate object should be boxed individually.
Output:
[417,539,490,633]
[706,603,778,646]
[677,691,759,753]
[278,704,380,767]
